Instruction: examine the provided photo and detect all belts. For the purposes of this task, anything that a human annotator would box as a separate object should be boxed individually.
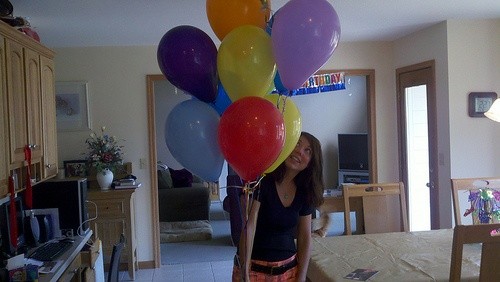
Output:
[234,255,298,275]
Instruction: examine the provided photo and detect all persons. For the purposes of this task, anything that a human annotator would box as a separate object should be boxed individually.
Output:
[230,132,324,282]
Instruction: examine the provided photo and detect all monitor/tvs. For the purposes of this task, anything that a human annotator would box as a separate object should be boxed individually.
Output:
[0,197,26,253]
[338,133,368,172]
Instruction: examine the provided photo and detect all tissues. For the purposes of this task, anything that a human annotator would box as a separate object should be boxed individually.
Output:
[7,253,26,282]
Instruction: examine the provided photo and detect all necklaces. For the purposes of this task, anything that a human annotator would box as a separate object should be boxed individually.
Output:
[284,194,288,199]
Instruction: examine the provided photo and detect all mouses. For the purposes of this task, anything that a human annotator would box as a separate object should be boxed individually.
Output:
[60,238,74,243]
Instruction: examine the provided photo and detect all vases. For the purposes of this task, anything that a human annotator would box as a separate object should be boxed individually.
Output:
[97,169,114,190]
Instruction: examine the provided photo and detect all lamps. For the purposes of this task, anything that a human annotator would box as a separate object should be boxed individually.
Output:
[483,97,500,123]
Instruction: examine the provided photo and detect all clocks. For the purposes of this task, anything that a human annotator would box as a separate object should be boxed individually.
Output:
[468,92,498,119]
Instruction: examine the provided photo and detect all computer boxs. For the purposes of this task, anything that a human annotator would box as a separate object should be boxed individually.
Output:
[32,178,90,236]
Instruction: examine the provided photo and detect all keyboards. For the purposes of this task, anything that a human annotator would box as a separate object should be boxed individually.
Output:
[28,243,69,261]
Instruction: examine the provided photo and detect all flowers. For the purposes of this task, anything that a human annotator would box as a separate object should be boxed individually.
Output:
[461,186,500,238]
[79,125,125,176]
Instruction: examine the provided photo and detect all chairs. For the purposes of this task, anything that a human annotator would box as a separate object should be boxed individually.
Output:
[451,177,500,226]
[341,181,408,236]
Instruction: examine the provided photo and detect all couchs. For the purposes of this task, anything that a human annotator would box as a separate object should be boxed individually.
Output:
[158,161,211,223]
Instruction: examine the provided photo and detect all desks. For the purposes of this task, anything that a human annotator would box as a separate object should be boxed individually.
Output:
[294,228,483,282]
[308,194,366,234]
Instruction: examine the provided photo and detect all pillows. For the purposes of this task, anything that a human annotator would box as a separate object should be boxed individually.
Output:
[158,220,213,243]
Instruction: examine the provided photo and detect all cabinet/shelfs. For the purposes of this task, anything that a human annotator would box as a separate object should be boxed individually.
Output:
[0,20,59,200]
[87,183,141,282]
[0,229,101,282]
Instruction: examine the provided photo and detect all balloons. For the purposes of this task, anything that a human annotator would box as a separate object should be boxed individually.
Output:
[267,12,296,95]
[164,100,226,182]
[216,24,278,101]
[272,0,342,94]
[263,94,302,174]
[156,25,217,102]
[193,82,232,116]
[218,96,287,185]
[206,0,271,40]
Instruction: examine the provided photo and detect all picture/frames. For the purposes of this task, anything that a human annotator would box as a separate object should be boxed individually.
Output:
[53,80,91,133]
